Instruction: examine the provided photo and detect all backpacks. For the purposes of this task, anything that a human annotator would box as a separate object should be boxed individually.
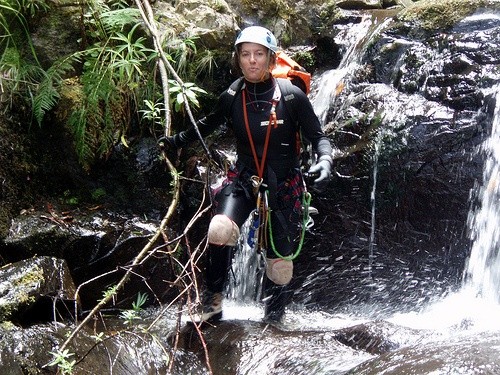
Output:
[217,51,311,172]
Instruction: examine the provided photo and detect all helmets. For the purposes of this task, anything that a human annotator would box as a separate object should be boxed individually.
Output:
[234,25,278,55]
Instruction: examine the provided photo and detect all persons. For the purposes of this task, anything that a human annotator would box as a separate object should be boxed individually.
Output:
[156,26,332,325]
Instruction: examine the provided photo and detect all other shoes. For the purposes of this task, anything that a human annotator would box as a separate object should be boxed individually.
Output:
[185,291,222,324]
[262,292,286,324]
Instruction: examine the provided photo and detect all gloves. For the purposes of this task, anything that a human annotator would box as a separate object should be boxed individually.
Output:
[308,157,333,184]
[156,133,182,154]
